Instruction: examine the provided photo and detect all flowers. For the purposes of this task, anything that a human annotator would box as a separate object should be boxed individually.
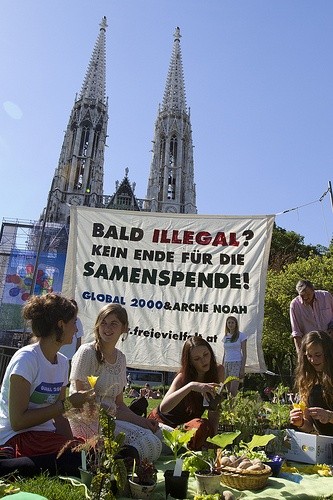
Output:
[87,375,100,394]
[292,401,308,416]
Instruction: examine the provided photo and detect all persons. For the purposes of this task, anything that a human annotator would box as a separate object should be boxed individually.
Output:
[289,331,333,437]
[0,280,333,485]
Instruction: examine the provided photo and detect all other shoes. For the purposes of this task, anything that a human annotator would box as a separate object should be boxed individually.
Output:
[0,447,14,458]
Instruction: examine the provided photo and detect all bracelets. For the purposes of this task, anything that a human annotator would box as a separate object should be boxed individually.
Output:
[61,399,73,414]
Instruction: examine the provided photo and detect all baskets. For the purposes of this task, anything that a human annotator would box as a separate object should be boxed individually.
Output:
[220,464,271,491]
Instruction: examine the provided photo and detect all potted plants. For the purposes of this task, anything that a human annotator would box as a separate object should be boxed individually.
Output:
[129,458,158,500]
[185,429,277,495]
[56,401,105,491]
[159,426,198,500]
[236,447,283,473]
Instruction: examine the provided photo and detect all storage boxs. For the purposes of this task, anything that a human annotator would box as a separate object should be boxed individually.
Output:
[285,430,333,465]
